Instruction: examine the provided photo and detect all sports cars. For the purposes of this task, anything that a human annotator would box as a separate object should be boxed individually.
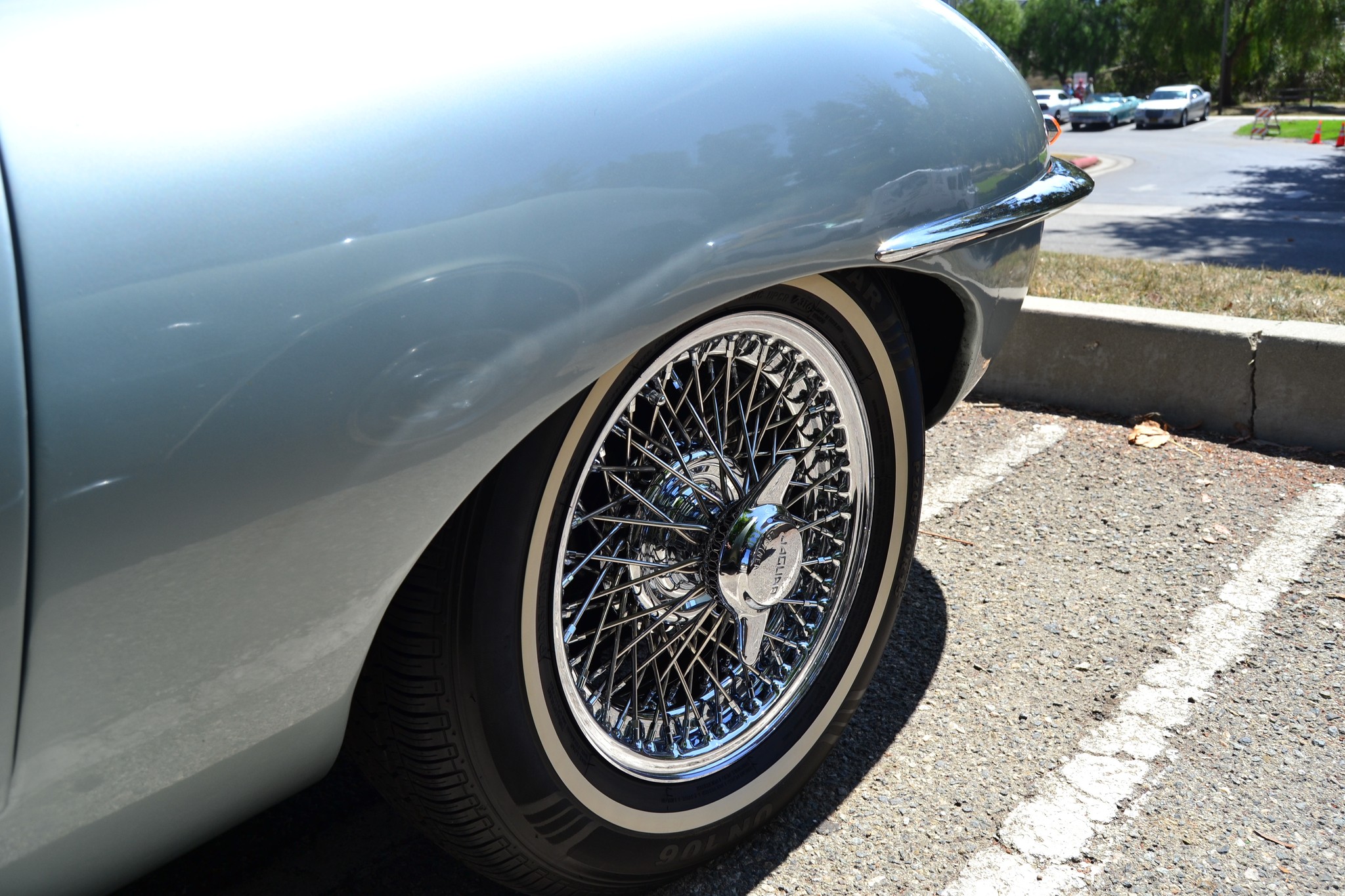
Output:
[1067,93,1144,133]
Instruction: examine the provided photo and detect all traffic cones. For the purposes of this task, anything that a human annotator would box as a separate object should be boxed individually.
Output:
[1305,121,1323,144]
[1336,122,1345,148]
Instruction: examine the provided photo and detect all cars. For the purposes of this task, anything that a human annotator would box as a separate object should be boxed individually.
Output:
[0,0,1102,896]
[1031,88,1082,130]
[1134,84,1212,131]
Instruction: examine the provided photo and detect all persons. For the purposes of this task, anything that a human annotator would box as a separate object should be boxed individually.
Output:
[1085,77,1094,103]
[1074,79,1084,104]
[1061,76,1074,97]
